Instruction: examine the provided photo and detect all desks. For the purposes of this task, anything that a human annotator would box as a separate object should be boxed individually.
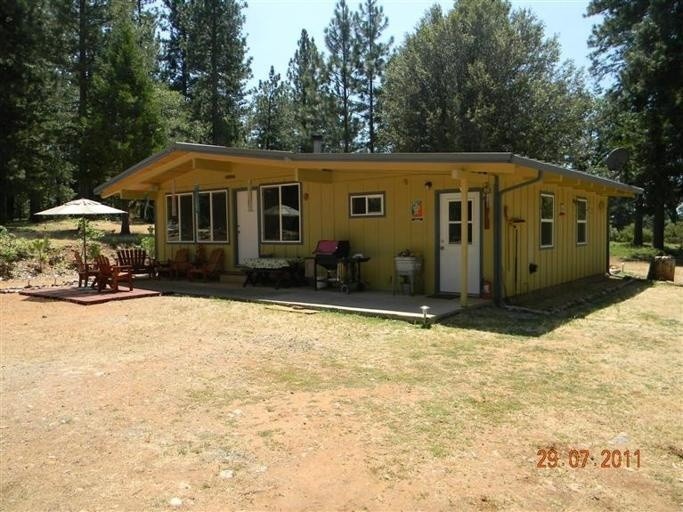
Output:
[304,256,369,293]
[239,258,302,288]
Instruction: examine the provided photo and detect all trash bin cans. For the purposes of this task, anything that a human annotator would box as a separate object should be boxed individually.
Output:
[312,240,351,291]
[655,256,676,282]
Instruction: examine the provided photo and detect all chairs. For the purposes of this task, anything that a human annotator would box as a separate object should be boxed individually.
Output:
[74,251,133,293]
[155,247,224,281]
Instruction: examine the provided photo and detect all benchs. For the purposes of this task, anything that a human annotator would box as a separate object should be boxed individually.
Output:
[108,250,156,279]
[241,268,299,290]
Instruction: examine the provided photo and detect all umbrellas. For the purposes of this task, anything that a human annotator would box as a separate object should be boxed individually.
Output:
[31,195,128,270]
[264,201,298,224]
